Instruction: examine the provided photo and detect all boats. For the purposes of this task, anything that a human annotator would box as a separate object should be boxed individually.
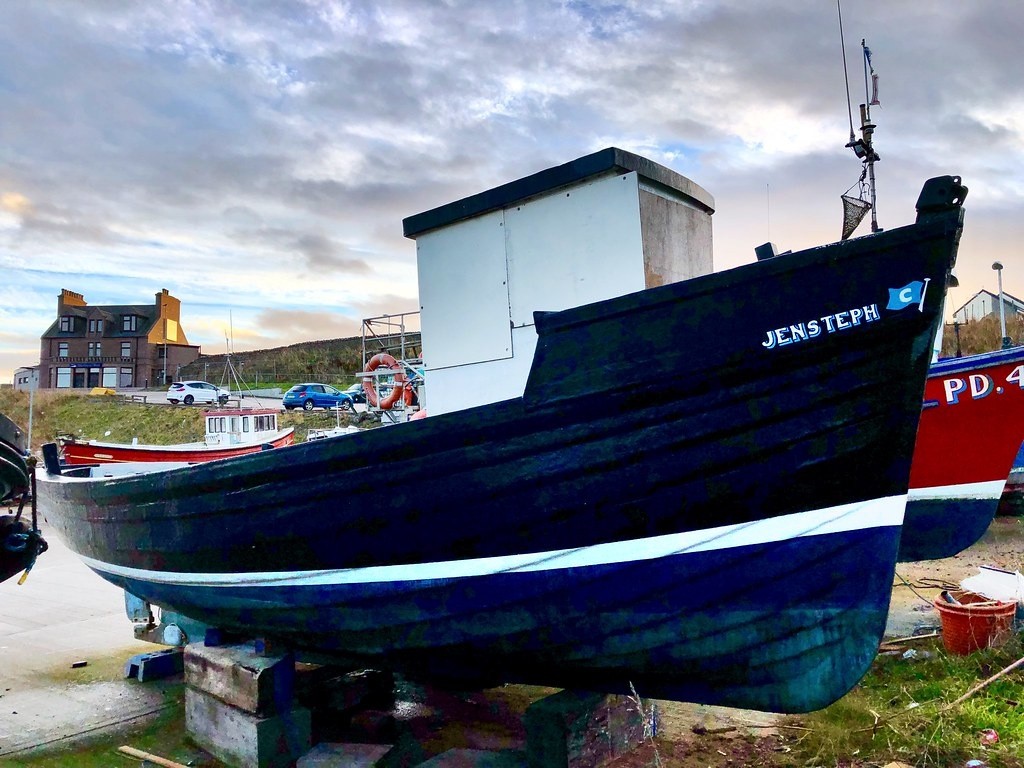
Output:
[32,0,971,714]
[897,345,1024,561]
[0,413,38,503]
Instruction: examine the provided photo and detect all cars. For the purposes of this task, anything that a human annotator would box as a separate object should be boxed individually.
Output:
[341,384,367,404]
[167,382,230,405]
[281,382,354,411]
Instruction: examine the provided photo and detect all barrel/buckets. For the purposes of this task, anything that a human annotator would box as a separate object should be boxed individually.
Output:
[934,590,1016,655]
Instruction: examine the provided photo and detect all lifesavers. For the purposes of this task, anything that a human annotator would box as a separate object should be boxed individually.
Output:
[362,354,403,409]
